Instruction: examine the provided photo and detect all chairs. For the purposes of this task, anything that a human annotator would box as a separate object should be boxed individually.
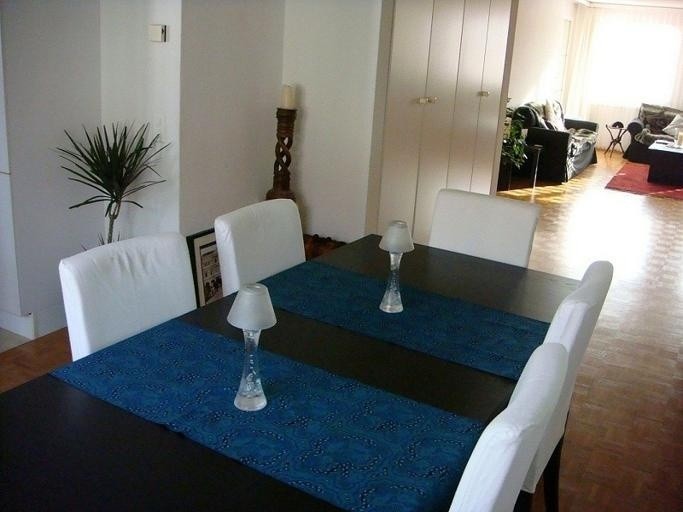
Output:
[448,260,614,512]
[623,102,682,164]
[427,189,541,269]
[59,233,197,361]
[214,199,306,296]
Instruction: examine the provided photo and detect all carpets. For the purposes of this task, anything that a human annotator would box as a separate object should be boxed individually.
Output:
[605,161,682,201]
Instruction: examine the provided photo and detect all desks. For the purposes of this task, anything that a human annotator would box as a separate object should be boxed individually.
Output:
[0,234,582,512]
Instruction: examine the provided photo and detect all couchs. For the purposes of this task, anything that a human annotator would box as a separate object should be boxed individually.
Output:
[511,99,599,183]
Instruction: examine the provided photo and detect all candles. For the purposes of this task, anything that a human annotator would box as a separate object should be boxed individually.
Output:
[280,84,296,109]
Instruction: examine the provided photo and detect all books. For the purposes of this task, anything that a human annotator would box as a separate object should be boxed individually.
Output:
[655,139,668,145]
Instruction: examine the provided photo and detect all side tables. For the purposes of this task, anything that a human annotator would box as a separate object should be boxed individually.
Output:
[604,124,629,159]
[523,141,544,189]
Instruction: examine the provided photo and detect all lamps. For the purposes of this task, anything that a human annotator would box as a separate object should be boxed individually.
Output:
[226,283,277,413]
[379,219,415,313]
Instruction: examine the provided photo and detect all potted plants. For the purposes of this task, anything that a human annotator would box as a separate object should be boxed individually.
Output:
[497,108,528,191]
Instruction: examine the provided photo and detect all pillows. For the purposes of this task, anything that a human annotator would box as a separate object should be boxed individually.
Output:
[661,112,682,137]
[543,97,563,132]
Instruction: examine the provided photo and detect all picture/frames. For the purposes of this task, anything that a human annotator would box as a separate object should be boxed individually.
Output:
[187,229,223,310]
